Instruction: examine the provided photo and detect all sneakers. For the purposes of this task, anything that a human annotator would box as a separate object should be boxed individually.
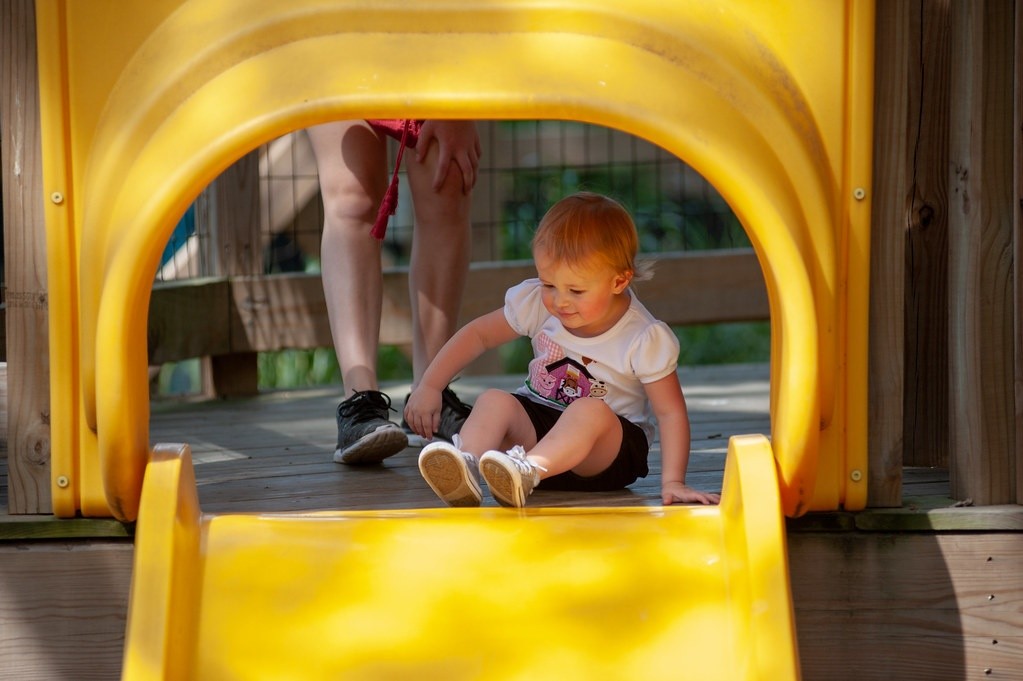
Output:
[400,375,471,447]
[334,387,407,466]
[418,434,484,508]
[477,444,548,508]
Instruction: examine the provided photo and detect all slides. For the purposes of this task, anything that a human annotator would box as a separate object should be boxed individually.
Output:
[119,430,799,681]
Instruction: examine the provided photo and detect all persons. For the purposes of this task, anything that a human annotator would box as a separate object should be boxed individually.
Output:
[307,119,473,462]
[404,193,721,508]
